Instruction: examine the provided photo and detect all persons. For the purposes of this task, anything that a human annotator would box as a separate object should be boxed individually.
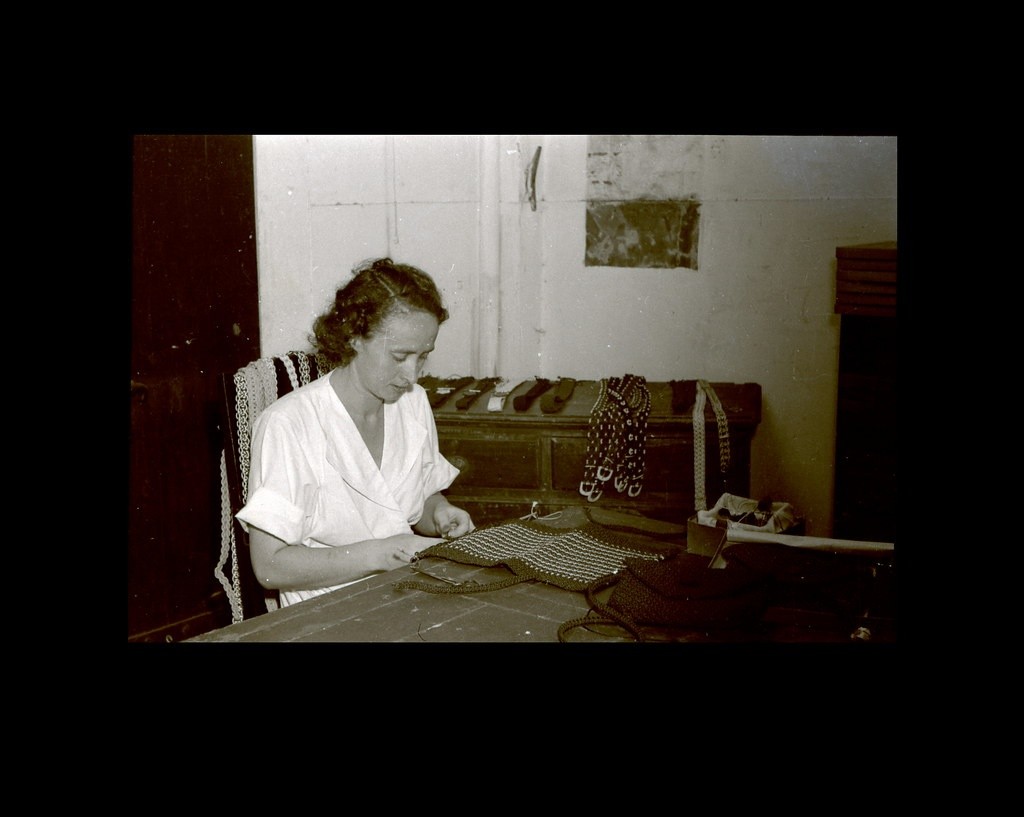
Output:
[234,258,475,609]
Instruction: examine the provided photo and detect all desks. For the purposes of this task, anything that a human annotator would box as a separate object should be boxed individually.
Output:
[182,508,691,643]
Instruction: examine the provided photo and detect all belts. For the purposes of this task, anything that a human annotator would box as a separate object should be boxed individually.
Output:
[455,377,503,411]
[514,376,554,413]
[699,379,731,486]
[692,382,707,511]
[430,376,474,408]
[580,374,651,502]
[486,377,526,412]
[541,376,578,413]
[421,375,439,391]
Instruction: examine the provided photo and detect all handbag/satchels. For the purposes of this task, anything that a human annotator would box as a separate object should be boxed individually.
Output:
[558,557,749,642]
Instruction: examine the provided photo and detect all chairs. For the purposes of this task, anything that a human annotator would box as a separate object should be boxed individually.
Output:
[216,353,332,620]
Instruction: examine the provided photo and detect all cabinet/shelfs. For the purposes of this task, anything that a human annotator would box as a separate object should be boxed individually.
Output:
[419,379,762,528]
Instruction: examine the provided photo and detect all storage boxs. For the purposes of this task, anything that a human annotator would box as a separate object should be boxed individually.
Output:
[687,494,793,559]
